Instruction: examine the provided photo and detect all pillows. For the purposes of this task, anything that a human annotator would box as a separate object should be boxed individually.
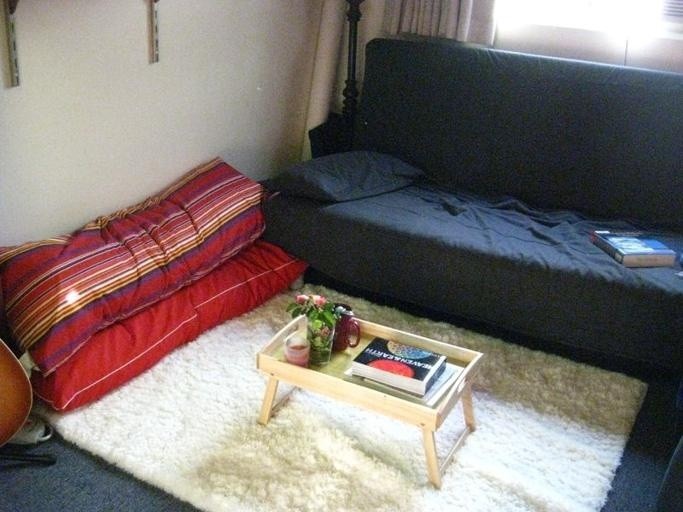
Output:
[31,240,308,413]
[0,157,266,375]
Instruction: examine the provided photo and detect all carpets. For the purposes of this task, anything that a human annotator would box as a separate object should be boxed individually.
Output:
[45,280,646,512]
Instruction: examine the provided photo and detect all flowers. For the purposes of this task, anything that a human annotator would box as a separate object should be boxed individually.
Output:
[285,295,340,357]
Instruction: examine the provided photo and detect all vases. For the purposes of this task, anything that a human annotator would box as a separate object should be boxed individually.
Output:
[309,322,334,365]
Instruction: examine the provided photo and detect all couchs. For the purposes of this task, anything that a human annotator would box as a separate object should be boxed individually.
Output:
[266,36,683,371]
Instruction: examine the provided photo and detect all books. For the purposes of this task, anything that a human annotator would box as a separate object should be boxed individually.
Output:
[592,228,677,268]
[343,337,465,408]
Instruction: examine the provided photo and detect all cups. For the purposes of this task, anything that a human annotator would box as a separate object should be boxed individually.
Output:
[334,304,361,353]
[285,335,310,367]
